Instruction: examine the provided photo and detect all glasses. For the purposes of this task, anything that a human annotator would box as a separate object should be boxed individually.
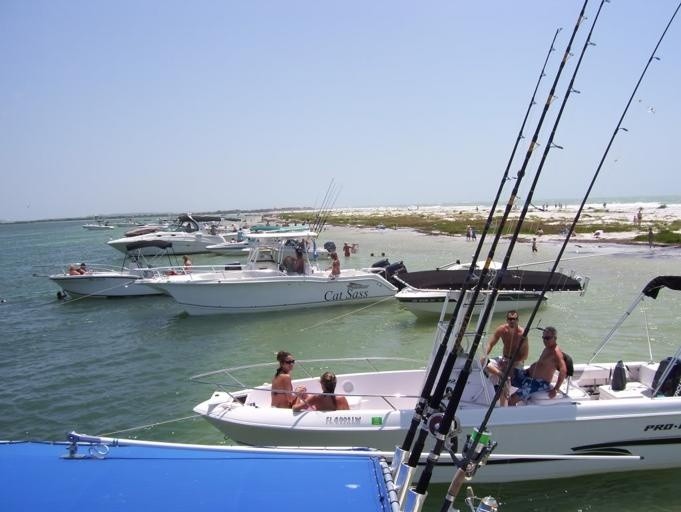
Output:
[542,336,553,339]
[507,318,518,320]
[286,360,294,364]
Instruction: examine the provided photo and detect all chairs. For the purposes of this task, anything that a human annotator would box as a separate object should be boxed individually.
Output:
[524,352,574,405]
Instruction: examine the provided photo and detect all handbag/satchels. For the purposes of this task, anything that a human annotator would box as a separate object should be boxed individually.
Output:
[612,361,626,390]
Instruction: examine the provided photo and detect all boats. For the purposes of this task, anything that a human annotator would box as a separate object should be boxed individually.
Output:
[192,361,681,484]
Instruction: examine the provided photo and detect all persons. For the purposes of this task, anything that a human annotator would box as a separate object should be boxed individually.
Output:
[267,217,311,229]
[183,254,193,273]
[467,225,476,241]
[209,224,245,244]
[293,373,349,412]
[508,326,567,408]
[69,265,83,274]
[78,263,88,272]
[542,202,565,211]
[634,210,654,249]
[480,310,528,407]
[533,226,575,252]
[57,291,68,300]
[271,351,305,408]
[324,240,356,279]
[284,253,307,274]
